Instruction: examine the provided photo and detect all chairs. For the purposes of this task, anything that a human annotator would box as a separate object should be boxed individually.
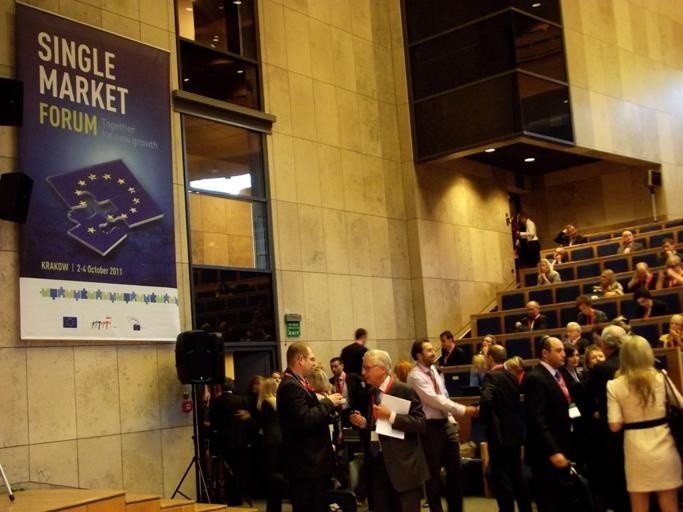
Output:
[195,282,277,340]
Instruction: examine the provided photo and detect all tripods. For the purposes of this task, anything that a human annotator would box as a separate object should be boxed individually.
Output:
[170,385,211,503]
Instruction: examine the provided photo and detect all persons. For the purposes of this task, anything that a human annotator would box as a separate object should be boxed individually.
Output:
[194,328,683,512]
[517,211,541,269]
[514,225,683,347]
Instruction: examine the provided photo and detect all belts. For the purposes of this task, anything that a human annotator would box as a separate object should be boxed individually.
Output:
[624,417,667,429]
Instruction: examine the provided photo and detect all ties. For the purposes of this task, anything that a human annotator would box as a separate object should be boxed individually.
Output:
[427,371,440,395]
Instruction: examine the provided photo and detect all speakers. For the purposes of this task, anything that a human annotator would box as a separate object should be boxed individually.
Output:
[647,170,661,187]
[175,330,225,384]
